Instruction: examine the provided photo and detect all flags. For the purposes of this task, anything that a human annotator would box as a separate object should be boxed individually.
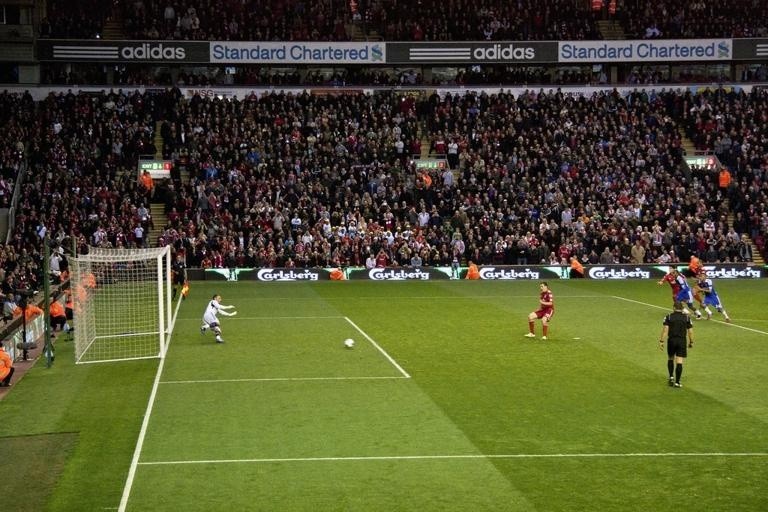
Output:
[180,282,190,298]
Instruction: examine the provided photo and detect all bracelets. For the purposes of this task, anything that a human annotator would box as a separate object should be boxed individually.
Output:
[660,340,664,343]
[689,341,693,344]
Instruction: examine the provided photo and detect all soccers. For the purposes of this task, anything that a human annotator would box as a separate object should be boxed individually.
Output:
[344,338,355,348]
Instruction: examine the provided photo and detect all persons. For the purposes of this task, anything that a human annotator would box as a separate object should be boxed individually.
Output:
[329,269,345,281]
[693,272,733,324]
[658,266,703,311]
[569,256,584,278]
[672,270,702,321]
[448,256,461,280]
[170,254,188,303]
[523,281,555,340]
[0,0,767,274]
[657,302,694,388]
[0,274,97,388]
[464,260,481,280]
[199,293,238,343]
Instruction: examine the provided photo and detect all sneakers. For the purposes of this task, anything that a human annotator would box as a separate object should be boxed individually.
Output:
[524,333,547,340]
[695,309,732,324]
[668,377,683,387]
[199,325,225,344]
